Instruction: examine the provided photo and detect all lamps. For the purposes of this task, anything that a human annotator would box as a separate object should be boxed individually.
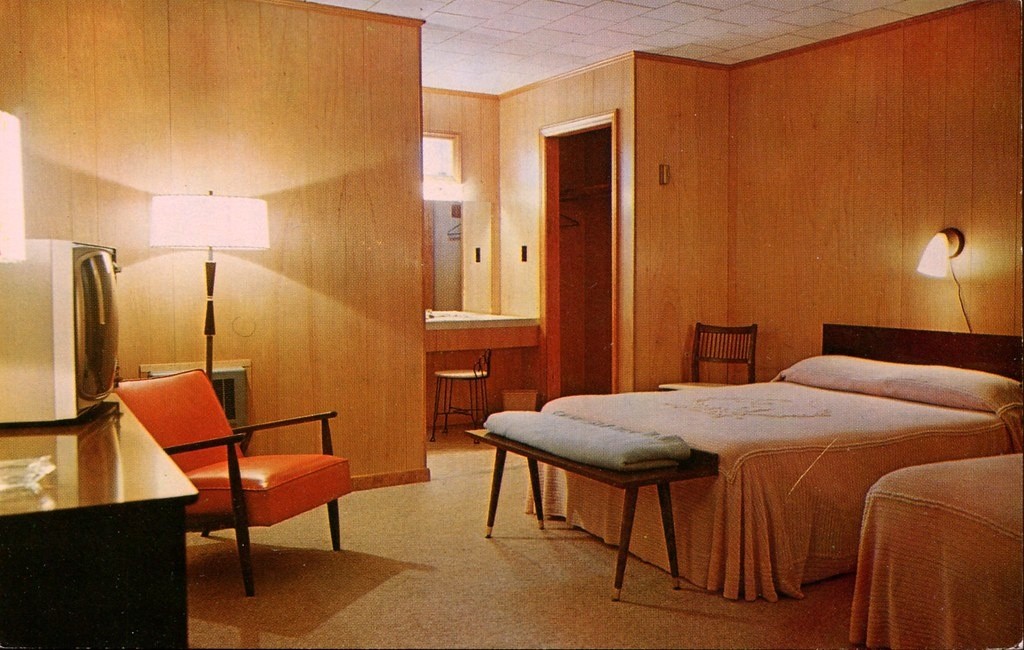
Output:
[147,193,273,391]
[917,228,965,278]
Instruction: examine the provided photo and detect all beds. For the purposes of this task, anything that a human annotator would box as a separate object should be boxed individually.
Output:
[847,454,1024,650]
[525,323,1024,604]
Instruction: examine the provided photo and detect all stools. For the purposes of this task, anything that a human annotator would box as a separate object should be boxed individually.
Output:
[428,348,493,445]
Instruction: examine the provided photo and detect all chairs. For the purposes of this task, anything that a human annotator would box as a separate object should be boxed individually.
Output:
[657,322,757,394]
[113,368,354,599]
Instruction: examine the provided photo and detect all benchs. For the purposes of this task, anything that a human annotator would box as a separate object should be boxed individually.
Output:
[463,409,717,602]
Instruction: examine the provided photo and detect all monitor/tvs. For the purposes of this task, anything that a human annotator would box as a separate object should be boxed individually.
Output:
[0,238,120,423]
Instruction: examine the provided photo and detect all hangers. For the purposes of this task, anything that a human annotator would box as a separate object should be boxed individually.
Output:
[446,223,460,236]
[560,213,579,228]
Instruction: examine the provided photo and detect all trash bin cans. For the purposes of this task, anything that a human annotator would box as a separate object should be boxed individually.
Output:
[501,389,539,412]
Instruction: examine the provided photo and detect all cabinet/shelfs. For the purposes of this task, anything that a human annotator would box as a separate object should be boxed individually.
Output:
[0,393,201,650]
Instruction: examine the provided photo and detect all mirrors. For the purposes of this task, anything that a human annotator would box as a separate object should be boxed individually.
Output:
[421,200,492,313]
